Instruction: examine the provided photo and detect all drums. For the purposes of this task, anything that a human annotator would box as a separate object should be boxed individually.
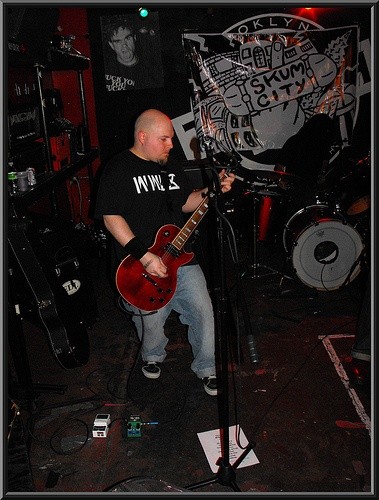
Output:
[281,204,365,293]
[238,187,282,244]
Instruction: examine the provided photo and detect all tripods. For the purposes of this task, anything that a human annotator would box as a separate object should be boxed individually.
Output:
[183,142,258,492]
[227,183,296,292]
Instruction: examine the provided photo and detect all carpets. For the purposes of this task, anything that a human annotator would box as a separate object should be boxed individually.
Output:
[319,332,371,438]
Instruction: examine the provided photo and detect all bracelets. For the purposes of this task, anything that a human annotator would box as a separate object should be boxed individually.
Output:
[123,237,149,260]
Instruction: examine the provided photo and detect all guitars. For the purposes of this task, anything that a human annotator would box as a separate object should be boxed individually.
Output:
[114,153,243,312]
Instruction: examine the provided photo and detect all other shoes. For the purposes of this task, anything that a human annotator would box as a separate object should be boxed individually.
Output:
[351,358,370,386]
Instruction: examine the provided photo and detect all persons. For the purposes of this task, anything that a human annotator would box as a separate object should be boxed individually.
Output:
[104,14,159,91]
[92,108,236,396]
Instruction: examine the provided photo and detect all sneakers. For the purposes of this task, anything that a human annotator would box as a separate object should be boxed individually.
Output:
[202,374,217,396]
[142,361,161,379]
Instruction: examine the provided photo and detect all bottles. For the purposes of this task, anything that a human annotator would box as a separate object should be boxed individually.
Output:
[7,162,18,196]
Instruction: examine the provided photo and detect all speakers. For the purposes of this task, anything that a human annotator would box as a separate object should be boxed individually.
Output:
[17,256,99,330]
